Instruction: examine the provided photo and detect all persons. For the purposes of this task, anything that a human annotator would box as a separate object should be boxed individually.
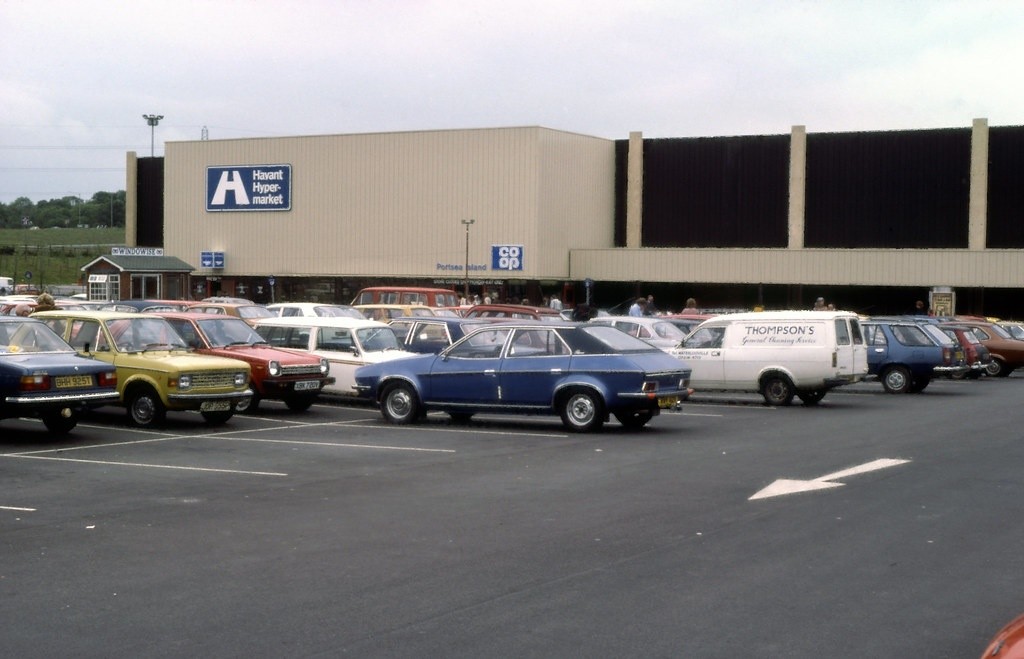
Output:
[457,294,926,317]
[682,298,699,315]
[15,293,66,337]
[629,297,646,317]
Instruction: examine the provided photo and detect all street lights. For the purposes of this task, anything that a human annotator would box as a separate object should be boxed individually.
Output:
[461,219,474,299]
[142,114,164,157]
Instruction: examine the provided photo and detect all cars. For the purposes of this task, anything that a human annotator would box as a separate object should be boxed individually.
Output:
[146,312,336,412]
[0,316,120,434]
[246,287,715,400]
[0,277,275,328]
[860,315,1024,394]
[28,310,254,429]
[351,320,694,433]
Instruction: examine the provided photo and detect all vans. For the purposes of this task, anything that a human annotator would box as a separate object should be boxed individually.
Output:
[661,310,869,406]
